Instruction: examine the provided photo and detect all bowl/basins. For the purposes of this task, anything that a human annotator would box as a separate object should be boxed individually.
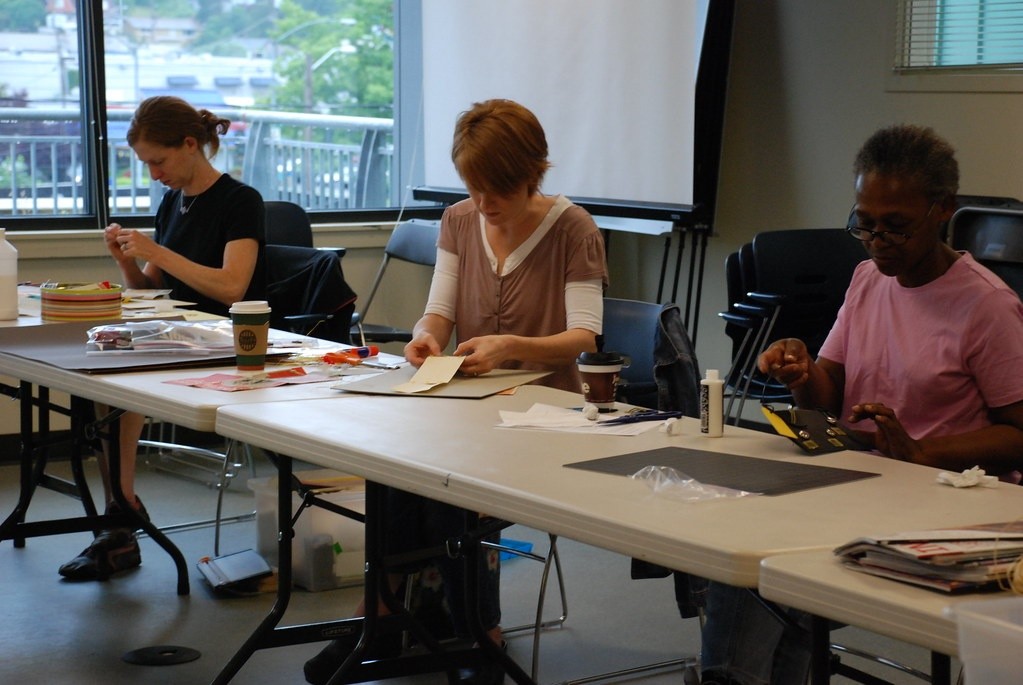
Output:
[40,282,122,323]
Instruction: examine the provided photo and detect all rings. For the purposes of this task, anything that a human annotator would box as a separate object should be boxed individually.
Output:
[120,244,125,251]
[474,372,477,376]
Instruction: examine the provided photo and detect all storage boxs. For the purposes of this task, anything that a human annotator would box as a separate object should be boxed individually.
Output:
[247,468,367,592]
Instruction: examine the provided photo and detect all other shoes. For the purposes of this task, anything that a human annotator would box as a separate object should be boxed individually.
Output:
[58,494,150,581]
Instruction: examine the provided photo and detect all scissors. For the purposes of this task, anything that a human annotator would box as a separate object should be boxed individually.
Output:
[325,351,389,370]
[596,409,682,425]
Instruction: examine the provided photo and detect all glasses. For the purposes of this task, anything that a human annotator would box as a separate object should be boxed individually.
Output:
[846,200,937,246]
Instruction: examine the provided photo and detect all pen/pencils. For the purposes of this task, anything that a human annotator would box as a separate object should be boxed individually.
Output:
[566,406,619,414]
[266,340,303,346]
[24,292,41,300]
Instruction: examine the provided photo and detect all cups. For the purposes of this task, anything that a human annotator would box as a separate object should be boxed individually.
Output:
[228,301,272,369]
[576,351,625,410]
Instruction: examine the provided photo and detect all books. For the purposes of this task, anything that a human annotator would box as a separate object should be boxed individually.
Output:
[833,521,1023,592]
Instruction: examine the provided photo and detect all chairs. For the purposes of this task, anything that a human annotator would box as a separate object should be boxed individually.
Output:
[453,295,703,685]
[264,199,366,348]
[713,194,1023,685]
[132,244,360,558]
[349,218,443,348]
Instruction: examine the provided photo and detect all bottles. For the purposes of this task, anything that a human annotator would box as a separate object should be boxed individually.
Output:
[0,228,19,321]
[699,369,725,437]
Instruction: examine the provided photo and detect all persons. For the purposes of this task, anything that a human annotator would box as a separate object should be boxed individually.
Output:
[700,124,1023,685]
[59,97,263,580]
[304,99,609,685]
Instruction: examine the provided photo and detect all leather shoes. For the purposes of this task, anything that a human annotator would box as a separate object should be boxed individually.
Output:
[304,613,403,685]
[459,640,509,685]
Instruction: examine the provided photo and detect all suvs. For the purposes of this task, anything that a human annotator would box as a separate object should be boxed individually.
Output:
[0,29,138,196]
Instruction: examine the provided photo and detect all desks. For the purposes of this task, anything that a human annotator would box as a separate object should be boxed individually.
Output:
[212,371,1023,685]
[0,280,407,685]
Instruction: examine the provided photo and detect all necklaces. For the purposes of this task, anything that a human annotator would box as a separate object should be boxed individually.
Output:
[180,169,213,214]
